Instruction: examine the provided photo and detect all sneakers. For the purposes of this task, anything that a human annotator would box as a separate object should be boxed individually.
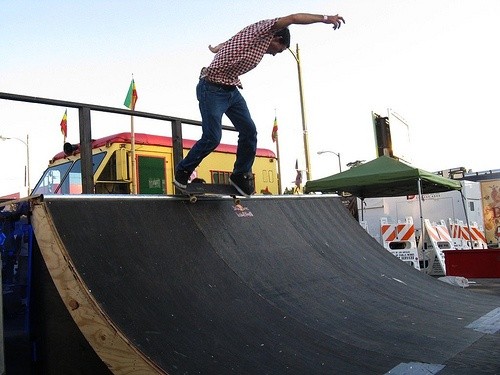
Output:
[174,168,191,189]
[230,172,255,197]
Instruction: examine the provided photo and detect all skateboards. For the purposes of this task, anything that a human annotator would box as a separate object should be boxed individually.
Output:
[171,181,243,205]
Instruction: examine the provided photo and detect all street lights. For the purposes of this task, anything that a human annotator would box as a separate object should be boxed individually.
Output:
[317,151,341,173]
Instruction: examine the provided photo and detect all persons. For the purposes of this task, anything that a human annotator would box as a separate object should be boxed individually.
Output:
[173,13,345,197]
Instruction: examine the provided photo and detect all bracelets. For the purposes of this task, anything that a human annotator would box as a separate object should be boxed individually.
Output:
[323,15,328,23]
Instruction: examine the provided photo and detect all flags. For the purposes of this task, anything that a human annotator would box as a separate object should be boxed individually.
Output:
[272,117,278,143]
[60,109,68,137]
[124,79,138,109]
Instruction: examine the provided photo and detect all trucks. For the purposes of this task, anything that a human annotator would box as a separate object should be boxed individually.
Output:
[30,132,279,195]
